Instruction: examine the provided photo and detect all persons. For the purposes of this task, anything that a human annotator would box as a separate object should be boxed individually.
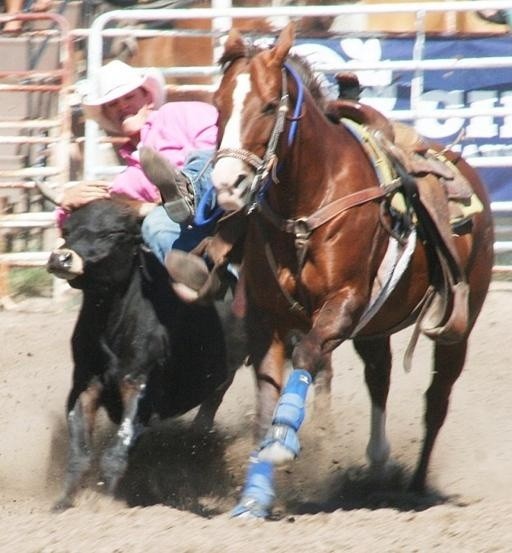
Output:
[56,58,231,300]
[2,0,55,35]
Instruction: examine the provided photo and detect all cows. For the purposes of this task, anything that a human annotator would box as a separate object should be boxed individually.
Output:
[33,177,261,515]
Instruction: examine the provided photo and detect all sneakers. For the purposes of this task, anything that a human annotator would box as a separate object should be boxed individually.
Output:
[165,249,237,304]
[138,146,195,224]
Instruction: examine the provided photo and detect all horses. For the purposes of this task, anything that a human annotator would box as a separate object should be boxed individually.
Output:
[125,1,332,106]
[208,19,495,524]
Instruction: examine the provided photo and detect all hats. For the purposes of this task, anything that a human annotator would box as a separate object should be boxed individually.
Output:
[76,60,167,135]
